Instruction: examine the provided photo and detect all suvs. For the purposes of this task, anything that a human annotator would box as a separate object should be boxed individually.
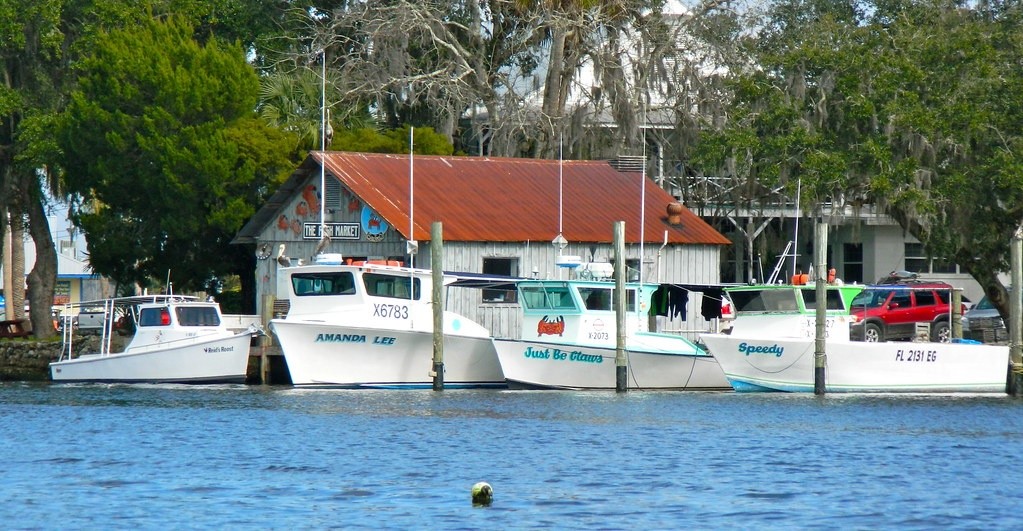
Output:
[849,271,966,343]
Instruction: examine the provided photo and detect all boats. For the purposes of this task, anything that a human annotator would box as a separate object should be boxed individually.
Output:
[49,269,263,385]
[697,179,1010,397]
[266,52,731,393]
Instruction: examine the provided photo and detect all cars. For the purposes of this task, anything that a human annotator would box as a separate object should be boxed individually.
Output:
[961,285,1012,333]
